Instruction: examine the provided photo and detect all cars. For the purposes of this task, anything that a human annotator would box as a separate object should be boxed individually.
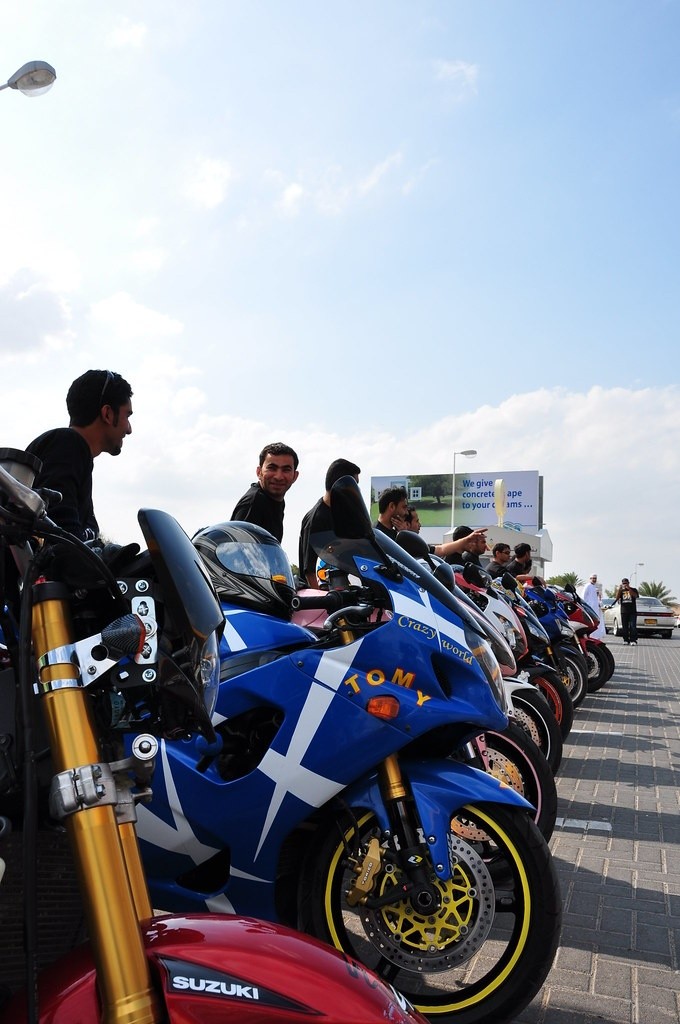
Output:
[674,615,680,628]
[600,598,616,607]
[604,596,674,639]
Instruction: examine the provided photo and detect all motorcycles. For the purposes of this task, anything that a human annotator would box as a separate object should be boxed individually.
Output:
[0,460,431,1024]
[414,553,588,779]
[550,583,615,692]
[58,508,562,1024]
[290,528,557,844]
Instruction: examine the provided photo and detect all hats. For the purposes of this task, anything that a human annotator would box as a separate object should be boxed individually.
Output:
[589,574,597,579]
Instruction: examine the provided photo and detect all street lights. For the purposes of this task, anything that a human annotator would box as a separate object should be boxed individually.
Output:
[451,450,478,529]
[635,563,644,592]
[630,572,636,586]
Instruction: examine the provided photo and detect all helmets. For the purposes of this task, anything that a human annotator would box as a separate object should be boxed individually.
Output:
[450,564,464,574]
[194,521,296,622]
[314,539,363,589]
[415,552,446,574]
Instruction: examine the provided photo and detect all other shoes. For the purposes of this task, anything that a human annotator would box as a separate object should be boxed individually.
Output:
[623,639,630,645]
[630,640,637,646]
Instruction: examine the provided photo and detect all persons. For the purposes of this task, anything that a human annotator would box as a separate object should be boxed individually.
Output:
[584,574,638,646]
[298,460,533,590]
[26,369,133,545]
[229,442,300,545]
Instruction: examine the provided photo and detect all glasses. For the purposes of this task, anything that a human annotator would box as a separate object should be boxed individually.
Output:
[622,583,628,585]
[97,368,115,407]
[498,550,510,556]
[590,578,597,579]
